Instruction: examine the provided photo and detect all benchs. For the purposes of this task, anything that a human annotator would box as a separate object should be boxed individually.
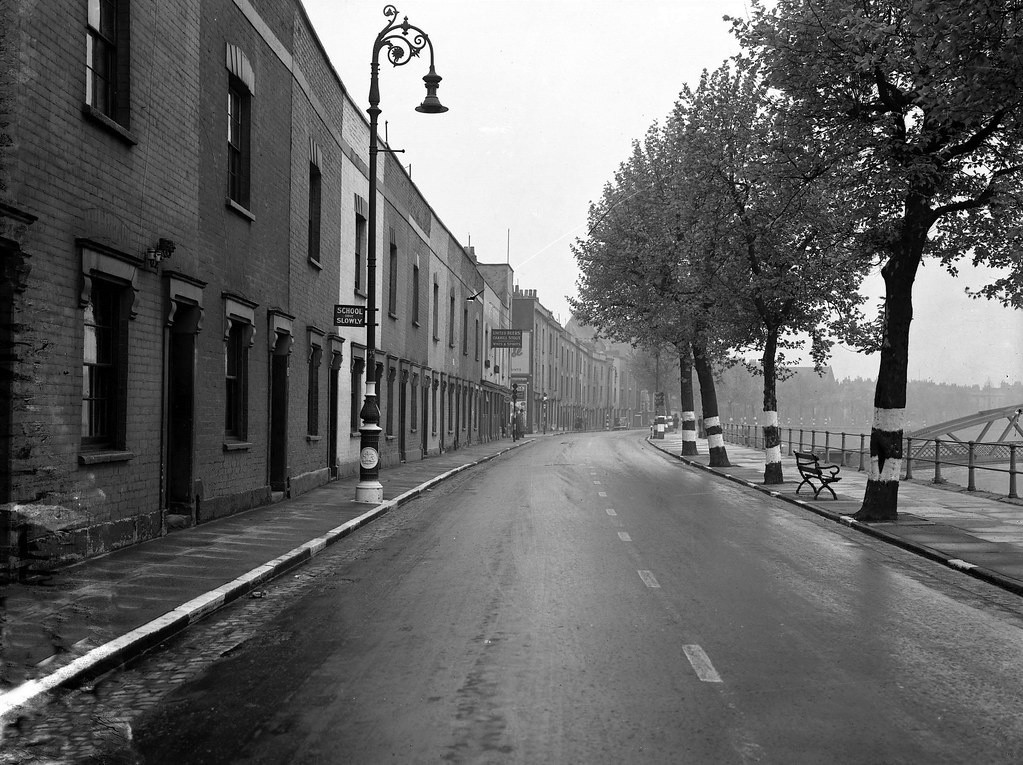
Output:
[792,450,842,501]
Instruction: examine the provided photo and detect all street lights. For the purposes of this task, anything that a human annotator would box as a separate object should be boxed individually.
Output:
[355,4,451,505]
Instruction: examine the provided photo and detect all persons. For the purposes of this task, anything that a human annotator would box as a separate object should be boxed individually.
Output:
[574,415,583,432]
[517,409,527,438]
[672,413,679,434]
